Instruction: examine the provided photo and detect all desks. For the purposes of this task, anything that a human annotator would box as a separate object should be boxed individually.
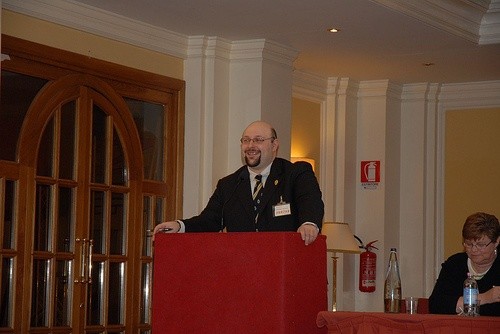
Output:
[316,310,500,334]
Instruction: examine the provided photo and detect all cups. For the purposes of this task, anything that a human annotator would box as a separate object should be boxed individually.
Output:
[405,296,418,315]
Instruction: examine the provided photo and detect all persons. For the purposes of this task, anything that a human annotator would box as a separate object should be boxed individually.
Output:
[430,212,500,317]
[153,120,324,245]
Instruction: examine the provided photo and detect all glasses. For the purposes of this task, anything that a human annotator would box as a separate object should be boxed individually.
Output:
[462,240,493,250]
[240,137,275,145]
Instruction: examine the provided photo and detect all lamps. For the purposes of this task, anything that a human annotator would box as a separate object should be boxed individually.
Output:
[321,221,361,311]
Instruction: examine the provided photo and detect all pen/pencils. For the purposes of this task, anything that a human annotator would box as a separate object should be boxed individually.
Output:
[146,228,173,231]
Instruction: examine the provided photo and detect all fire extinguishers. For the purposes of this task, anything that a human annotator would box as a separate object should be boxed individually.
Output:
[353,234,379,293]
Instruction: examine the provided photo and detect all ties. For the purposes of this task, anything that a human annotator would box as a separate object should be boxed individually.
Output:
[252,174,263,223]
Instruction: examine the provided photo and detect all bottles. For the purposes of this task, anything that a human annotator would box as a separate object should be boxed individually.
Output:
[384,248,401,313]
[463,273,478,316]
